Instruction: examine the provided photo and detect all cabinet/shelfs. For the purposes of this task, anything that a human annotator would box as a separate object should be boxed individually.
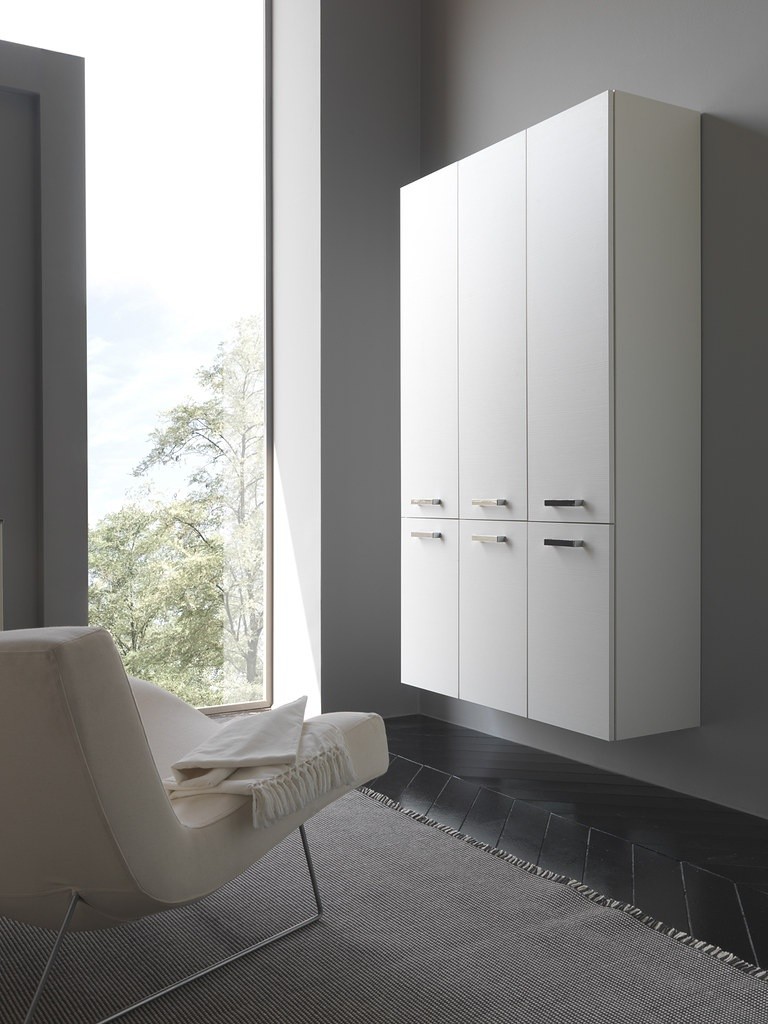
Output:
[400,89,699,743]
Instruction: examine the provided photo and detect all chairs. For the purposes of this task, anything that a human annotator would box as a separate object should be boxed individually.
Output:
[0,625,391,1024]
